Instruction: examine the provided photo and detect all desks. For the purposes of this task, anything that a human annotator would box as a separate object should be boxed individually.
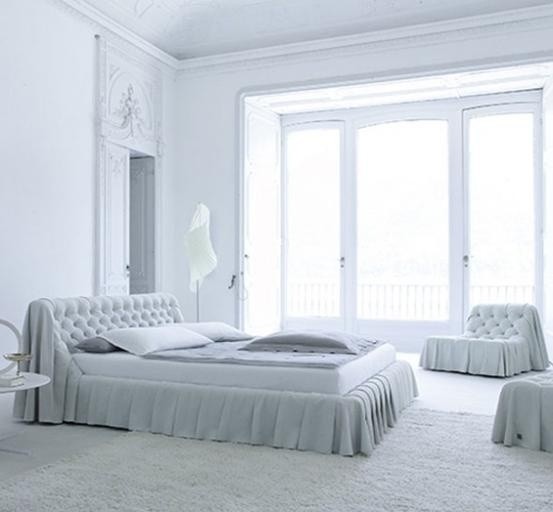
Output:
[1,372,51,456]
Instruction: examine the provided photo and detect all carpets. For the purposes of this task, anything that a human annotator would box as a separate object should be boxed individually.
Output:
[0,407,553,512]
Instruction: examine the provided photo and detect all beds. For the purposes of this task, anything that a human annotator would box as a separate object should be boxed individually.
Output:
[13,295,418,459]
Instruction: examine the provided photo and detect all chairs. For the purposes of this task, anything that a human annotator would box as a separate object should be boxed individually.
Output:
[492,374,553,454]
[419,304,549,377]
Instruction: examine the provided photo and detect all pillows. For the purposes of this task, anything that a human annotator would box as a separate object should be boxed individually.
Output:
[174,321,253,342]
[98,324,214,356]
[74,337,119,354]
[236,329,377,355]
